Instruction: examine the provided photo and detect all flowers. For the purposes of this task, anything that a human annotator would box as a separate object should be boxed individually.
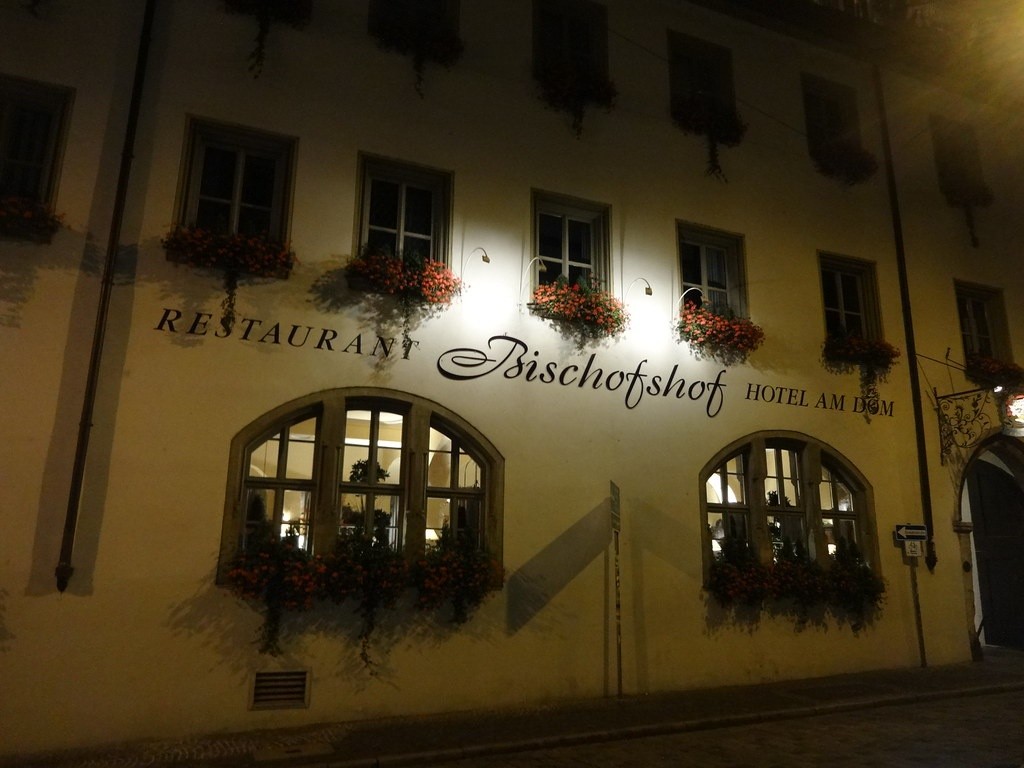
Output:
[964,348,1024,431]
[819,324,901,414]
[345,240,471,359]
[524,275,632,351]
[674,300,767,364]
[218,516,506,663]
[160,221,302,334]
[703,557,887,631]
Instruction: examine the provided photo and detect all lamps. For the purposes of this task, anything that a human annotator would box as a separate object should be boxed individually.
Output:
[462,247,490,279]
[934,383,1004,466]
[624,278,653,304]
[677,287,709,305]
